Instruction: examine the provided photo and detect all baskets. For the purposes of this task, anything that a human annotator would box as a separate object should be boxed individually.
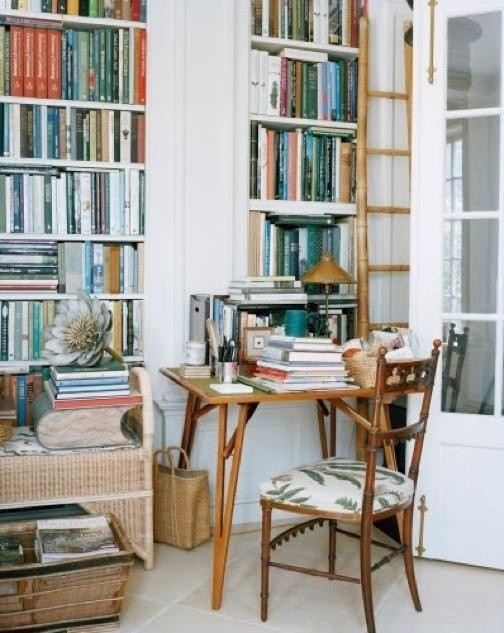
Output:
[0,559,134,628]
[1,418,13,442]
[342,355,422,389]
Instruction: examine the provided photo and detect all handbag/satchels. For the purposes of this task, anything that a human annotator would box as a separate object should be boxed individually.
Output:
[151,447,211,549]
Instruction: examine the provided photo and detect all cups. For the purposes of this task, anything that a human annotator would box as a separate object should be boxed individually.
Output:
[284,309,322,338]
[184,342,206,365]
[215,360,239,384]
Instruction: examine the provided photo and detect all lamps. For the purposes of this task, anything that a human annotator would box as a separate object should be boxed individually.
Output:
[298,255,357,336]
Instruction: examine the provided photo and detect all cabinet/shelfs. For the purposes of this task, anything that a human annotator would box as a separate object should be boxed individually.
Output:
[0,0,154,574]
[249,0,372,348]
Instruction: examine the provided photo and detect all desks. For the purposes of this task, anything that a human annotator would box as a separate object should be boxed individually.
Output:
[158,367,415,610]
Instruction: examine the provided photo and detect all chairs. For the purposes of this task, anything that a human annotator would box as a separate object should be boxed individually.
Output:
[257,339,442,633]
[442,323,470,413]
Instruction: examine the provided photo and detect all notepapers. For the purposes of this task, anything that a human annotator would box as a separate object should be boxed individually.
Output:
[210,383,254,395]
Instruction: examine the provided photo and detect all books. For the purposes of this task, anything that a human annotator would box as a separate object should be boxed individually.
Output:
[0,0,147,23]
[210,276,355,393]
[0,17,146,105]
[249,121,356,204]
[0,367,50,427]
[44,365,142,410]
[251,0,358,47]
[0,166,144,235]
[1,300,55,361]
[261,214,349,295]
[0,238,144,295]
[99,300,142,356]
[32,517,119,564]
[251,47,358,123]
[0,102,145,163]
